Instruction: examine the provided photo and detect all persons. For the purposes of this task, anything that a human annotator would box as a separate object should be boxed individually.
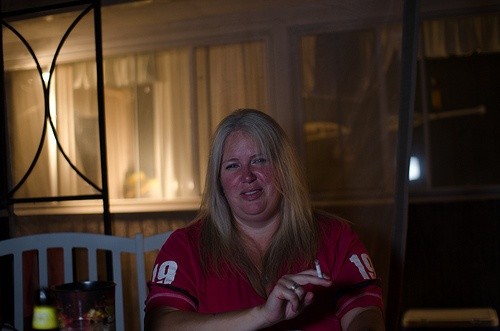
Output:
[143,109,385,331]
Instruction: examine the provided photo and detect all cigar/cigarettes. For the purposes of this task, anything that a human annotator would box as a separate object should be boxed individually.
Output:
[314,260,321,277]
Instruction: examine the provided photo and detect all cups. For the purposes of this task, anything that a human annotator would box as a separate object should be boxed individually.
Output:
[58,280,117,331]
[32,286,58,331]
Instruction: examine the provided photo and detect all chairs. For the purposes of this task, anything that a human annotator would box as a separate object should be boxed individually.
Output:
[0,231,173,331]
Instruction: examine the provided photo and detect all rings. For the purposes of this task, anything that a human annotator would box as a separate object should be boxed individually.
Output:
[290,283,299,290]
